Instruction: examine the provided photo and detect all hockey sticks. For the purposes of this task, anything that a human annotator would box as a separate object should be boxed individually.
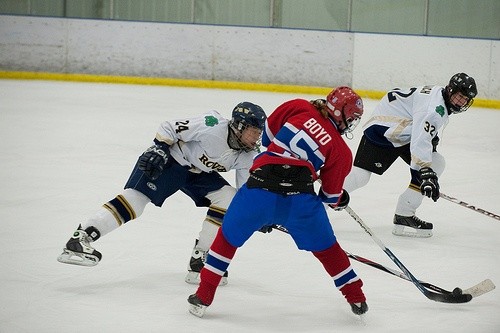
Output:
[267,222,496,300]
[435,188,500,222]
[341,201,473,304]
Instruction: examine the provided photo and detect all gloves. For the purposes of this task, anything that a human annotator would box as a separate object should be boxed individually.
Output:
[258,224,278,233]
[418,166,440,202]
[328,188,349,211]
[137,144,170,181]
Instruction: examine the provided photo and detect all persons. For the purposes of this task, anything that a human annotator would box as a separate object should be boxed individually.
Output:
[187,86,369,324]
[56,102,273,286]
[342,73,478,238]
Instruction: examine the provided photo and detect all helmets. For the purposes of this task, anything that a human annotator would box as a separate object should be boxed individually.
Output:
[324,86,365,132]
[232,102,267,133]
[447,72,478,115]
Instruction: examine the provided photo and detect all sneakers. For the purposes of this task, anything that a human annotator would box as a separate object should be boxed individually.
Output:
[57,224,102,266]
[349,300,368,326]
[392,210,434,238]
[187,294,206,317]
[185,251,228,286]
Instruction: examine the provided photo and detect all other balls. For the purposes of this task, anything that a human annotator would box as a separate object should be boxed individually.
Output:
[453,287,463,295]
